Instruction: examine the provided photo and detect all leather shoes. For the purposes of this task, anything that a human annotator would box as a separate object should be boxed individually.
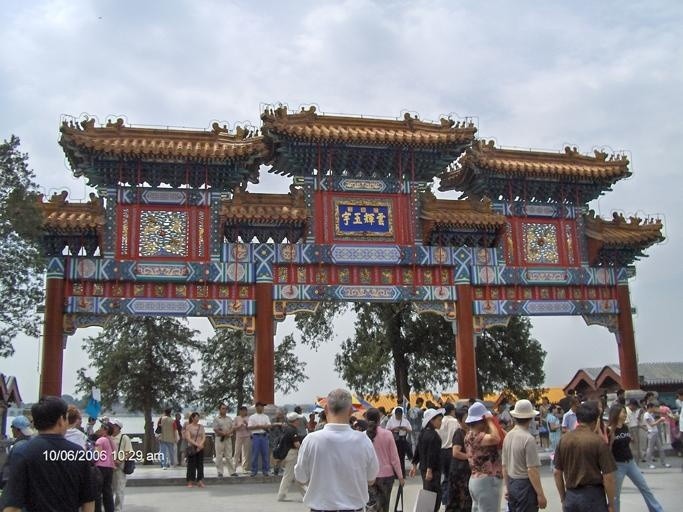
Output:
[250,472,270,478]
[218,473,239,478]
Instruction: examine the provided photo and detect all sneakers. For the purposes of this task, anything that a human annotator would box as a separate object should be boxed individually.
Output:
[647,463,671,470]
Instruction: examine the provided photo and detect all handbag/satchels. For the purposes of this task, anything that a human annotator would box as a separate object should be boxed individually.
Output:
[186,447,196,457]
[124,460,135,474]
[492,416,505,449]
[414,488,437,512]
[155,418,162,434]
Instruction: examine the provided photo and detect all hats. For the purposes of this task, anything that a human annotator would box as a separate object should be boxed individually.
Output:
[422,399,540,429]
[98,419,123,430]
[287,406,303,421]
[255,402,267,407]
[11,416,34,436]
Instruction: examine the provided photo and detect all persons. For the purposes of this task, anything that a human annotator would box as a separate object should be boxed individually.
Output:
[408,407,443,512]
[360,406,407,512]
[499,397,548,511]
[460,400,504,512]
[0,391,683,493]
[91,421,117,512]
[443,404,474,511]
[109,418,133,512]
[604,403,666,511]
[291,387,380,511]
[551,397,618,512]
[0,395,98,511]
[274,412,308,502]
